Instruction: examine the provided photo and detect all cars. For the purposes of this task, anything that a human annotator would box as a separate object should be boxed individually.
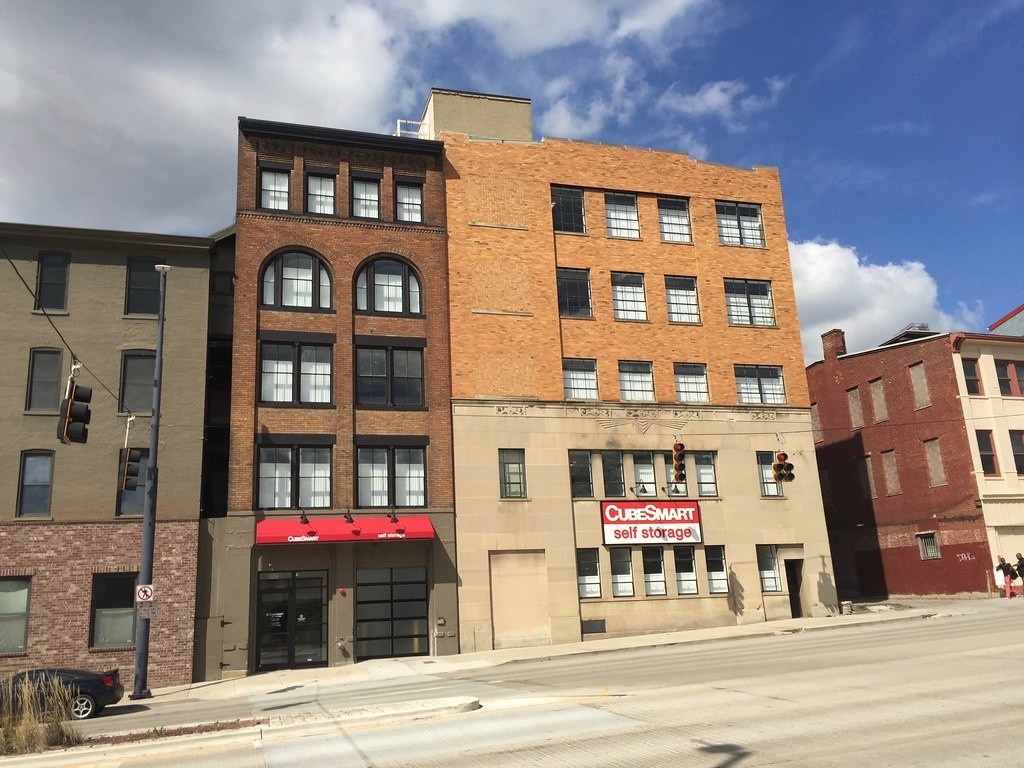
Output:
[0,668,124,720]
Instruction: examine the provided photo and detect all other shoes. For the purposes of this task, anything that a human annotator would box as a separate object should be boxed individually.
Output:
[1004,597,1011,600]
[1015,594,1020,598]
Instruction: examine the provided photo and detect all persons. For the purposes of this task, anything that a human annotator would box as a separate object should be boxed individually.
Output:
[996,558,1020,599]
[1012,553,1024,585]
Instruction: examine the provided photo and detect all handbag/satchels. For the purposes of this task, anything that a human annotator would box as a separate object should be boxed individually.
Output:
[1008,566,1018,580]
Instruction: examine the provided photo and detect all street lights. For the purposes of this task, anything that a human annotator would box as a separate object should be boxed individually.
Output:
[129,264,173,699]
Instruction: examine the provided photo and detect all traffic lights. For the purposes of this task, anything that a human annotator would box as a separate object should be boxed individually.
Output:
[772,452,795,482]
[118,448,141,492]
[57,377,92,445]
[672,439,685,482]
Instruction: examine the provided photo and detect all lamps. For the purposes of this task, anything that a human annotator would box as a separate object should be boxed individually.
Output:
[661,479,680,493]
[630,479,647,493]
[300,509,309,524]
[344,509,354,523]
[387,509,399,523]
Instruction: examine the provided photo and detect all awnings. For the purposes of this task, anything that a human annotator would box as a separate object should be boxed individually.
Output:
[255,516,435,544]
